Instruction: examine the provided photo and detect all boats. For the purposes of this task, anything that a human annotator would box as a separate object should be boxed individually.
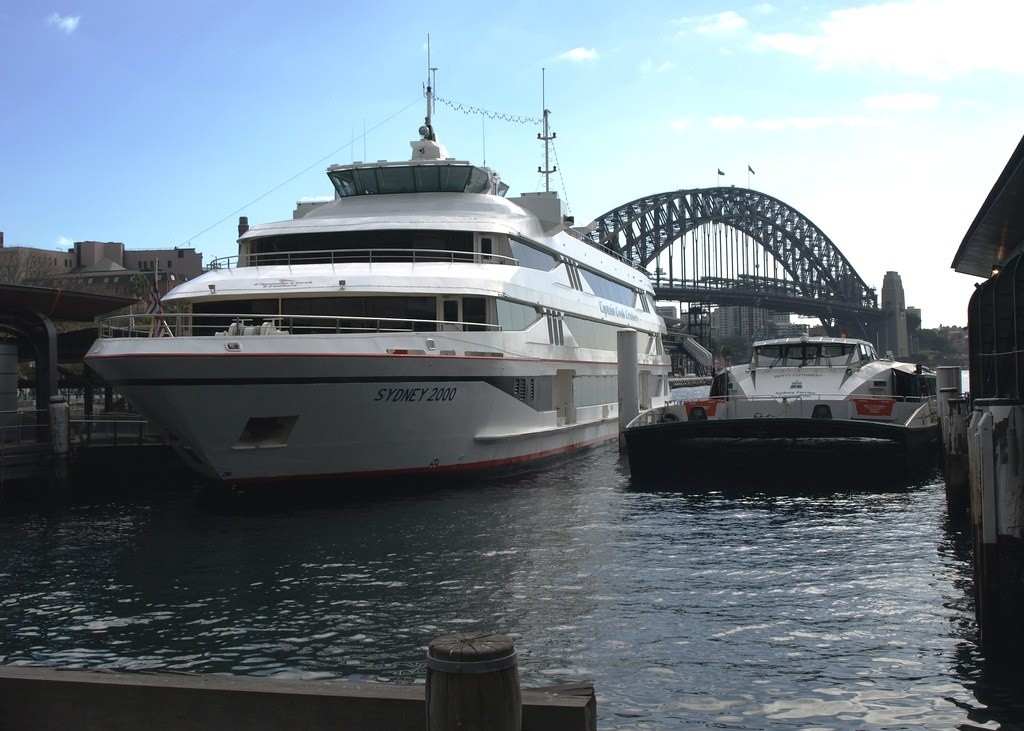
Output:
[620,332,939,491]
[83,30,673,507]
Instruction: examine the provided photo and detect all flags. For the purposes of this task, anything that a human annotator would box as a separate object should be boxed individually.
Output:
[718,168,725,175]
[146,277,163,323]
[749,165,755,174]
